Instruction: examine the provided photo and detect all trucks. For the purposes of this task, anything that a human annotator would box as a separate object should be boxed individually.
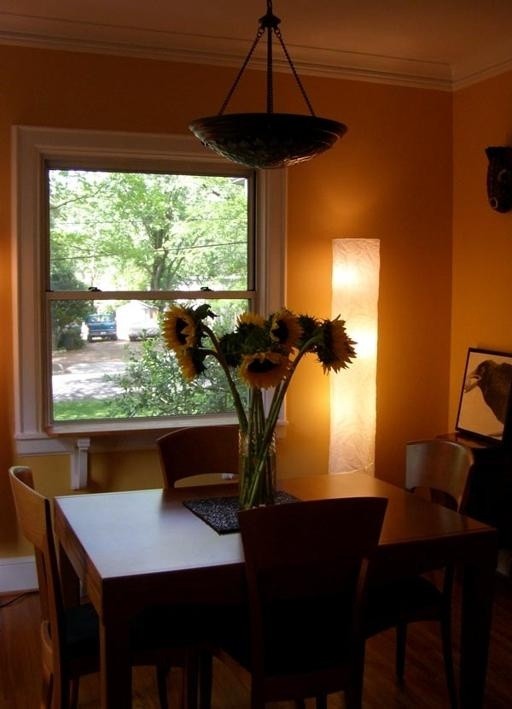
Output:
[86,313,117,341]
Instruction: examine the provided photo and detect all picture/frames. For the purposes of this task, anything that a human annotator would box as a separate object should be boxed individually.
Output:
[456,343,512,452]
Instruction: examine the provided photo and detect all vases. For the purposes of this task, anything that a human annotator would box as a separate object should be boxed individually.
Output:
[236,429,278,514]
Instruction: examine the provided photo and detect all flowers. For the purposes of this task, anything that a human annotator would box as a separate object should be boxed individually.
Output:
[157,299,356,512]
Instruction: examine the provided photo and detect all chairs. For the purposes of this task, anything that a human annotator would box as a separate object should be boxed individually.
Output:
[6,467,204,707]
[391,435,476,703]
[155,421,308,494]
[178,498,390,708]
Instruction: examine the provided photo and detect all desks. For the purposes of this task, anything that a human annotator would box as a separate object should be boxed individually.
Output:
[47,468,490,707]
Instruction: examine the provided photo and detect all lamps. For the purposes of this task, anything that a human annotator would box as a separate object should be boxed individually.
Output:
[187,1,345,175]
[327,235,380,479]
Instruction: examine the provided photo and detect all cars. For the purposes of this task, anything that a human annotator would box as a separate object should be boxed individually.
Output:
[127,321,160,340]
